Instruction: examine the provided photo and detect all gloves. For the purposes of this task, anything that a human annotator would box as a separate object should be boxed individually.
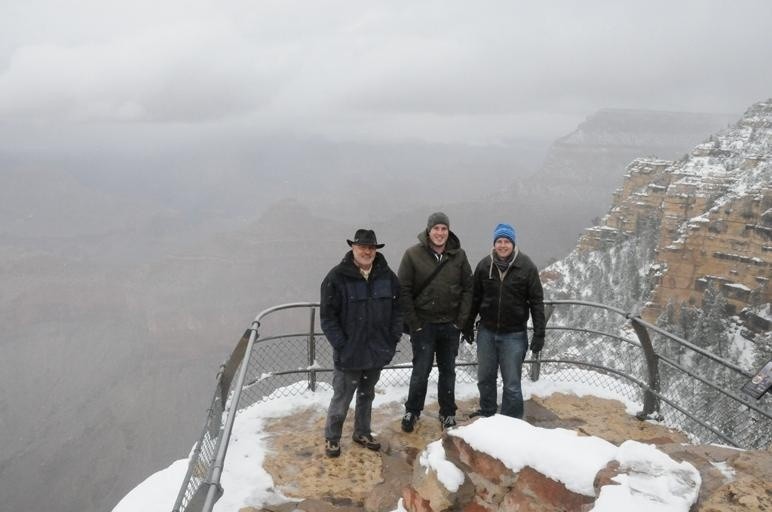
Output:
[461,322,475,344]
[529,334,545,354]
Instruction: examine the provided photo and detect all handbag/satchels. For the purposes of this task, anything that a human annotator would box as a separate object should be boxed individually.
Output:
[401,322,410,336]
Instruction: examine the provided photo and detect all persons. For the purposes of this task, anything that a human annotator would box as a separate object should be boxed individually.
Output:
[461,224,546,418]
[320,228,403,458]
[398,212,475,432]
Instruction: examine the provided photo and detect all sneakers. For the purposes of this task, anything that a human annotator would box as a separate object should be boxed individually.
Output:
[324,438,342,458]
[468,408,492,420]
[438,414,457,432]
[352,429,382,451]
[400,410,419,433]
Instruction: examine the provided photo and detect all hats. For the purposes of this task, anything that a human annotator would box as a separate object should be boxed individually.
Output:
[425,211,450,235]
[492,223,516,249]
[345,228,385,250]
